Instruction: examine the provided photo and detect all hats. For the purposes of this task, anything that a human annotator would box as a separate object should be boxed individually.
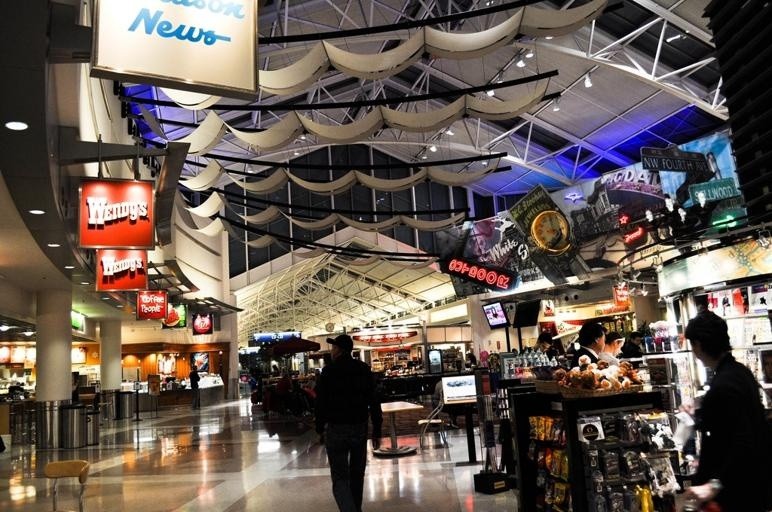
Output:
[327,335,352,350]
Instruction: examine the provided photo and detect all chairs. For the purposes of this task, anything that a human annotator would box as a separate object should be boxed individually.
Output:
[416,392,452,451]
[41,458,95,512]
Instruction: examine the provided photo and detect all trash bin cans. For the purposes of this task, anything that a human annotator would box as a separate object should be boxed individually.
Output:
[59,404,87,449]
[85,410,100,445]
[119,391,133,419]
[102,388,120,419]
[34,398,70,449]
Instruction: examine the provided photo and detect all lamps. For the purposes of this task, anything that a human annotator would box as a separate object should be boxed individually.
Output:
[417,29,625,174]
[639,189,713,252]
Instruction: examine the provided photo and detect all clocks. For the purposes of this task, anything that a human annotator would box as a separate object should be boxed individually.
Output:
[531,210,572,255]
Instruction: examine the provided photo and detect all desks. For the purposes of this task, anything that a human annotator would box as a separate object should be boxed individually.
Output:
[371,400,423,461]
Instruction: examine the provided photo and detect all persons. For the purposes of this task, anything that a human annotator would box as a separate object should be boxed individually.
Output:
[682,311,770,512]
[479,351,491,367]
[191,413,202,454]
[272,365,280,377]
[248,371,259,393]
[314,332,384,512]
[521,321,644,370]
[190,364,203,409]
[465,352,477,368]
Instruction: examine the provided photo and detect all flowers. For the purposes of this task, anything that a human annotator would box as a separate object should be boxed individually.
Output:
[550,354,646,391]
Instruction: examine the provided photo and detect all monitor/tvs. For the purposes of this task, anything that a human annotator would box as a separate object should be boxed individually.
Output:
[482,301,510,329]
[512,299,541,328]
[166,376,176,383]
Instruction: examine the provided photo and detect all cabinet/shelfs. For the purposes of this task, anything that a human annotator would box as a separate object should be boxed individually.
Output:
[370,346,412,375]
[669,278,772,403]
